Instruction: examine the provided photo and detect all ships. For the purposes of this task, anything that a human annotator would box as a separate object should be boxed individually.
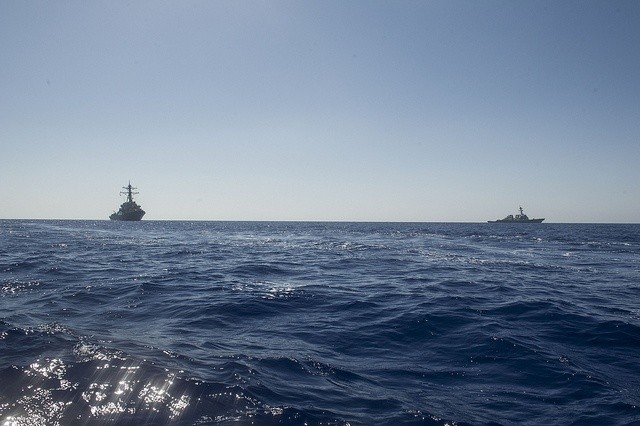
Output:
[488,205,545,223]
[108,178,146,221]
